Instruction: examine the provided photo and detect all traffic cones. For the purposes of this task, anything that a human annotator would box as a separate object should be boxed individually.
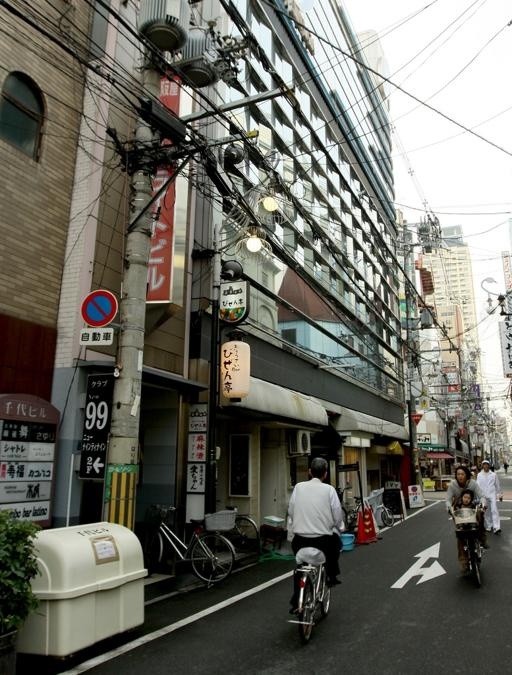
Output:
[355,508,383,545]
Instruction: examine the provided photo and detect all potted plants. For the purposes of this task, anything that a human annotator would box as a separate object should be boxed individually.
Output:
[0,507,43,675]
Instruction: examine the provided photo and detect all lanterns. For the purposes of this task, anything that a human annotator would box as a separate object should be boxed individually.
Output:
[220,335,250,402]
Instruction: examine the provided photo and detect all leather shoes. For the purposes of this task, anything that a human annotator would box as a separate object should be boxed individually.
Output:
[326,574,341,587]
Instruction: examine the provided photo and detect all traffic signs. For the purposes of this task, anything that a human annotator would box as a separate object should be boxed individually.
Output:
[78,372,116,483]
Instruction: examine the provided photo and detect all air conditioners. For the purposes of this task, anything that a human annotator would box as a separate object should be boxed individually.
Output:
[287,429,312,459]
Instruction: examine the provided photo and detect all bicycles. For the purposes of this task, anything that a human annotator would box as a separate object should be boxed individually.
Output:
[446,503,488,588]
[223,504,259,553]
[138,504,237,590]
[346,488,395,532]
[286,547,331,644]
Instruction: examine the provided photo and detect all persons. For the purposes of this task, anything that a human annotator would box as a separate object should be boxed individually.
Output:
[446,466,490,578]
[448,489,482,531]
[504,464,509,474]
[476,460,503,535]
[287,457,345,615]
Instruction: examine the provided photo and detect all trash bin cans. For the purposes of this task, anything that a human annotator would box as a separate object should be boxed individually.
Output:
[14,519,150,659]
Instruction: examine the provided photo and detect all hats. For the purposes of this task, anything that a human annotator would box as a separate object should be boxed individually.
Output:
[482,460,489,464]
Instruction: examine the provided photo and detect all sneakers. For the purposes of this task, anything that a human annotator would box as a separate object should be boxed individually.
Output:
[494,528,501,534]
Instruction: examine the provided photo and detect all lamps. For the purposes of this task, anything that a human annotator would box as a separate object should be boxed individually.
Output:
[234,226,272,265]
